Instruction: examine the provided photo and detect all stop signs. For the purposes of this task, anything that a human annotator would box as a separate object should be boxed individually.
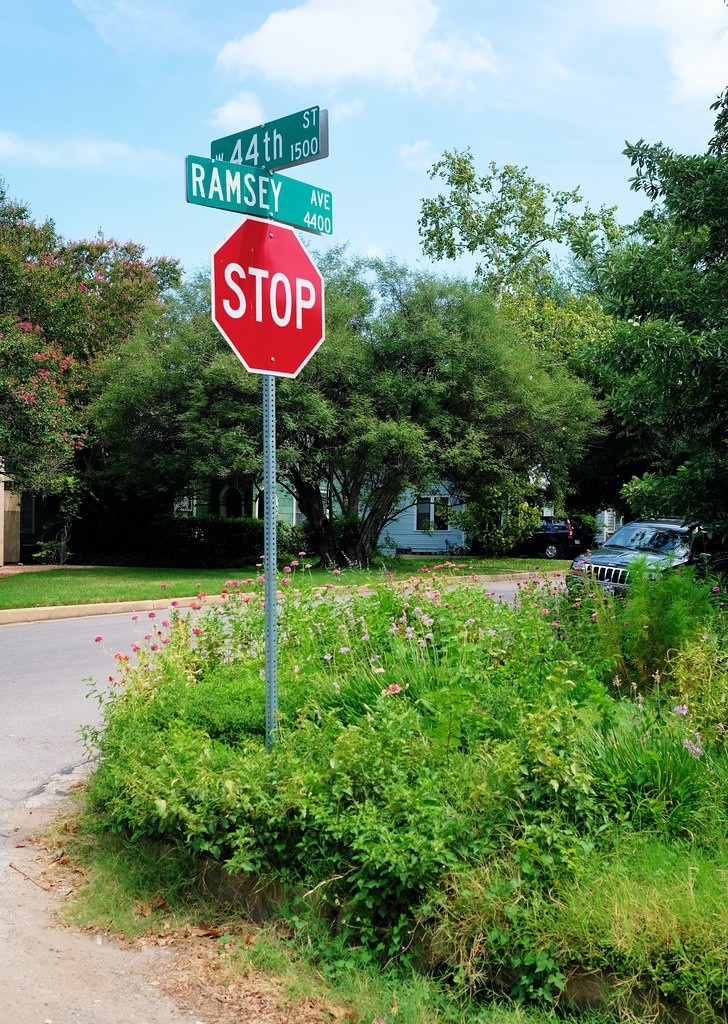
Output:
[210,217,327,378]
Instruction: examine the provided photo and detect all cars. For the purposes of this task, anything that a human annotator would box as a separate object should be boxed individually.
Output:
[505,517,600,558]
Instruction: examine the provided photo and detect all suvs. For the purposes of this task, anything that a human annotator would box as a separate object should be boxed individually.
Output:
[567,518,712,602]
